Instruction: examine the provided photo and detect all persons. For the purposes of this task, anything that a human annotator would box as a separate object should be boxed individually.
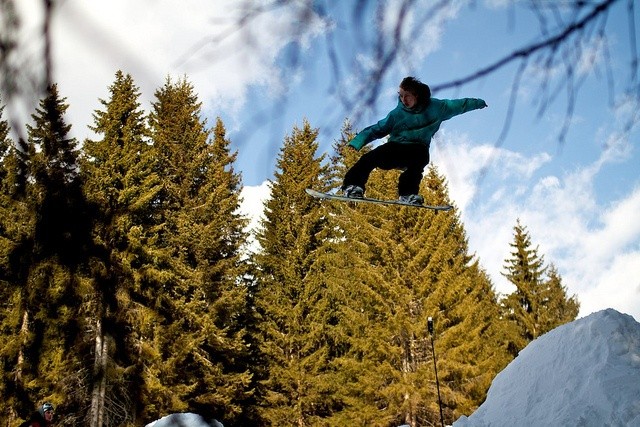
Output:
[340,76,489,205]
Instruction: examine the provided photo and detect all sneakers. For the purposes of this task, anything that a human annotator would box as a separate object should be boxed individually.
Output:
[344,185,364,199]
[399,195,425,205]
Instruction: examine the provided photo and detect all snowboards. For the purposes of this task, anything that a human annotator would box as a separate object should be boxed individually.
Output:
[305,187,455,214]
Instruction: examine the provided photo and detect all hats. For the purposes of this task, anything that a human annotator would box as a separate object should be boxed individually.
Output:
[42,403,54,412]
